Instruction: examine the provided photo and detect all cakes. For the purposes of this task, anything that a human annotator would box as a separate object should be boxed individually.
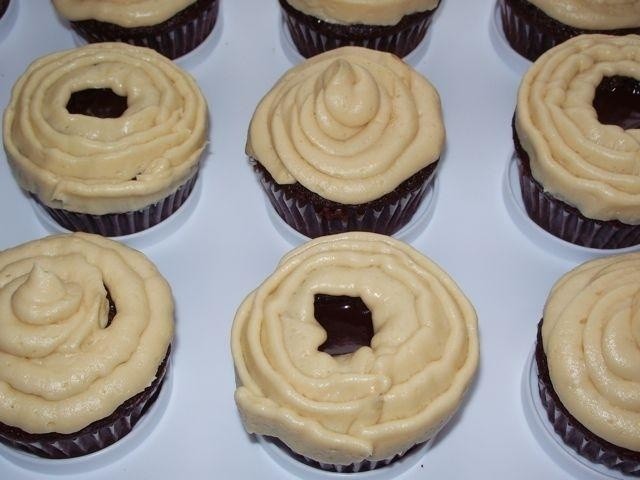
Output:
[511,33,640,251]
[499,0,640,65]
[0,230,174,460]
[245,45,447,239]
[231,230,480,472]
[53,0,219,61]
[277,0,442,59]
[2,42,209,238]
[534,251,640,478]
